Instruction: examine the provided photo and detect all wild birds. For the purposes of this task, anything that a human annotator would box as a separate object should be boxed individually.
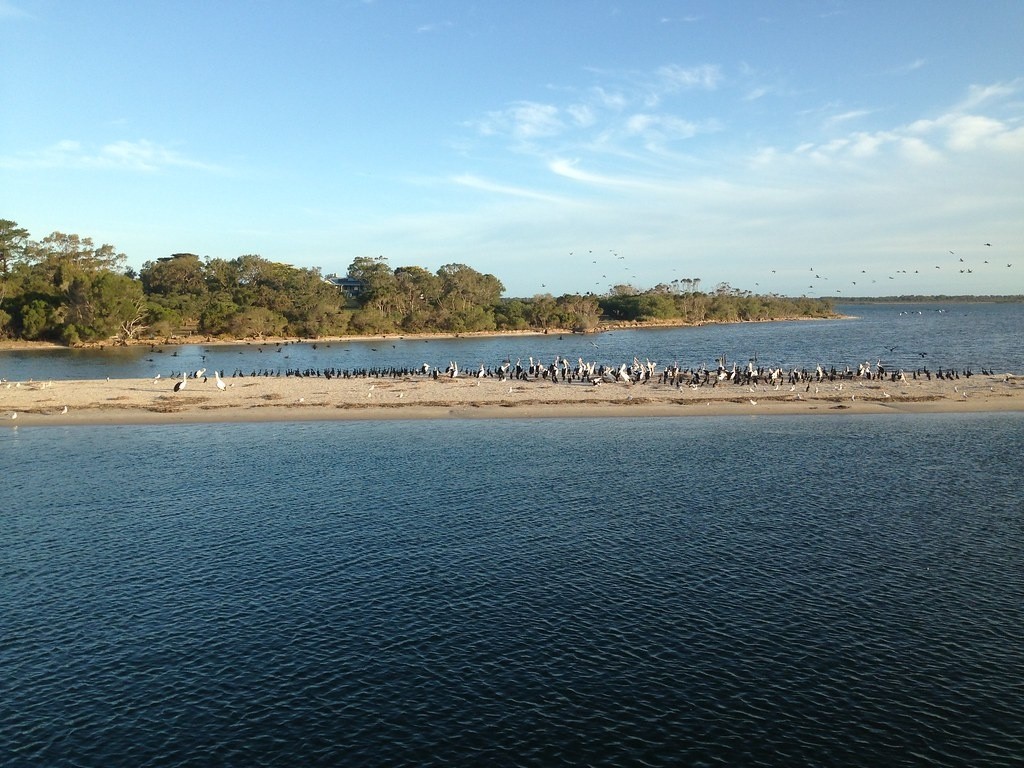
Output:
[0,242,1013,421]
